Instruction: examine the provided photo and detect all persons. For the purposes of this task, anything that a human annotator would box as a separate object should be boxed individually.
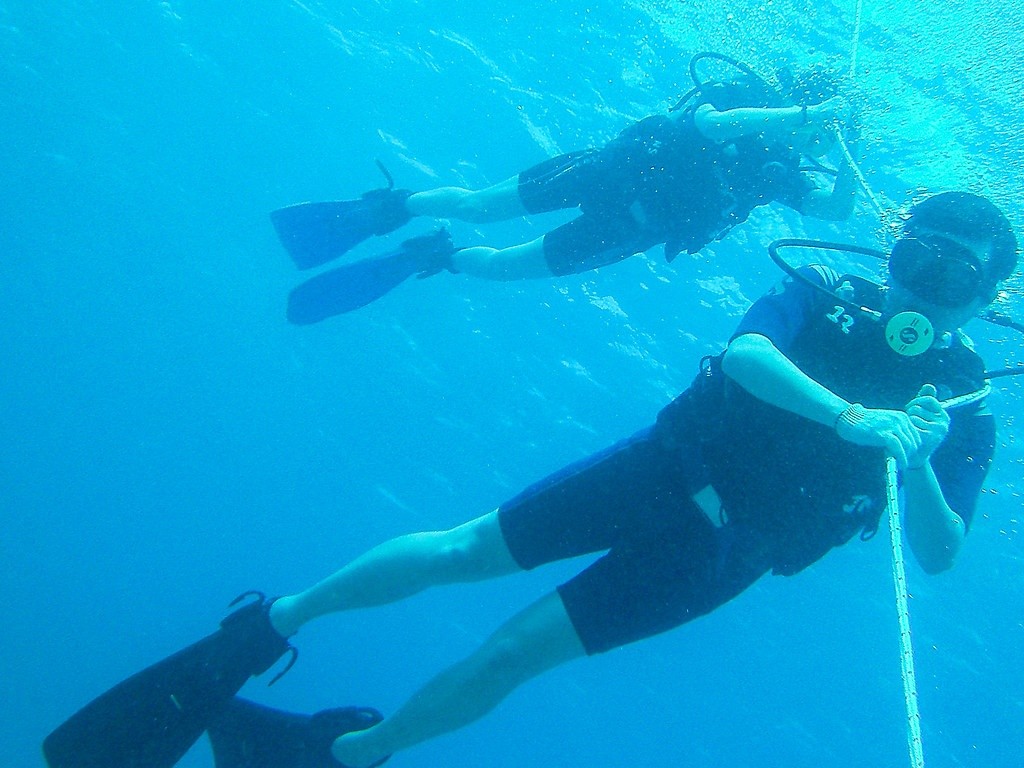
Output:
[44,191,1017,768]
[268,69,863,328]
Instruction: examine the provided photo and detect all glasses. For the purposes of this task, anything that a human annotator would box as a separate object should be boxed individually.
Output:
[886,237,982,308]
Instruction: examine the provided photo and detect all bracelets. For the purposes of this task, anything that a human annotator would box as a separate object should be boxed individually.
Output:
[802,102,808,126]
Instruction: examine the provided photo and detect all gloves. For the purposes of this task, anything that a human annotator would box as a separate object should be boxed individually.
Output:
[835,403,921,470]
[903,384,951,469]
